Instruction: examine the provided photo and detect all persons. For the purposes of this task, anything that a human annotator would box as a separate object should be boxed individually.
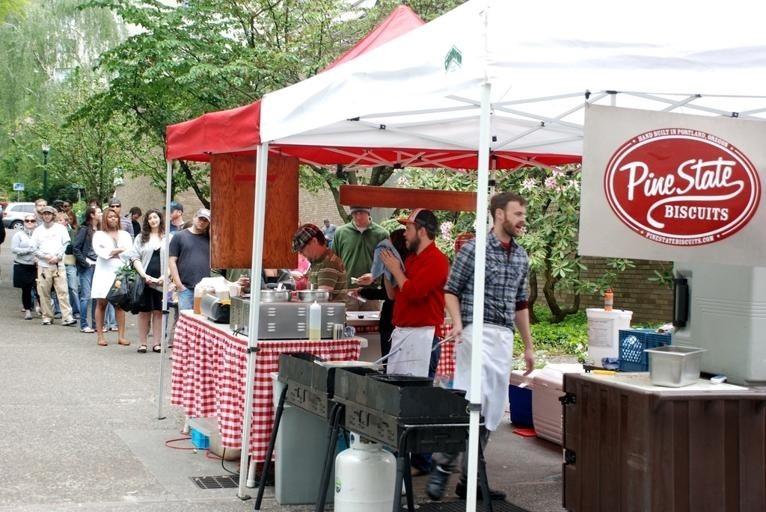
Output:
[420,192,537,500]
[320,220,337,250]
[345,229,412,374]
[1,198,310,352]
[394,205,452,382]
[331,201,386,311]
[291,222,345,302]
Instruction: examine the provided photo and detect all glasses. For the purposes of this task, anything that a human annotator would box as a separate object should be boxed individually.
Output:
[25,219,36,224]
[110,205,120,208]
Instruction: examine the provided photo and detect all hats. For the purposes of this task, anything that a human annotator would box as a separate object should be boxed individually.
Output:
[292,223,320,253]
[350,206,371,215]
[41,205,55,214]
[397,207,437,231]
[197,209,211,224]
[162,201,183,212]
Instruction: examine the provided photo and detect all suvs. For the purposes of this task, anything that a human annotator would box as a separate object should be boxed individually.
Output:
[2,202,39,232]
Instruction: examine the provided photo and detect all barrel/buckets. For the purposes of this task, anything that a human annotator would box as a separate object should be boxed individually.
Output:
[586,307,634,364]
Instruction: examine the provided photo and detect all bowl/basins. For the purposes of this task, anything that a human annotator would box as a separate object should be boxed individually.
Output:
[298,292,331,301]
[260,289,290,301]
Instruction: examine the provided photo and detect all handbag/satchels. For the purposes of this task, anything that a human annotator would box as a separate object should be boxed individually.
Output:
[106,267,147,311]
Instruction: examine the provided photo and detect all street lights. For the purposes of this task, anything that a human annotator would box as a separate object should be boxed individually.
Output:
[41,143,51,199]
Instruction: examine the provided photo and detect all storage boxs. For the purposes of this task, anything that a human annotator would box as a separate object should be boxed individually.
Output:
[532,370,578,447]
[508,369,542,428]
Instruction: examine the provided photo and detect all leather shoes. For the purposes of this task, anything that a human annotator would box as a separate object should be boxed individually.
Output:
[137,344,147,354]
[152,344,162,353]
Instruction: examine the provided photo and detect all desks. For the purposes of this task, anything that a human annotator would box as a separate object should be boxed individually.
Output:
[558,372,766,512]
[343,311,456,380]
[169,310,369,488]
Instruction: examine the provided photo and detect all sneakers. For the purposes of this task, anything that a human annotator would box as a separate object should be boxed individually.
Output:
[424,465,452,503]
[97,338,107,347]
[118,337,130,346]
[21,307,119,332]
[454,475,507,502]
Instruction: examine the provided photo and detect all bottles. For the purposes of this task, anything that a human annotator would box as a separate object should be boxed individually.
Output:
[334,323,344,340]
[309,300,321,340]
[193,283,201,314]
[604,288,614,312]
